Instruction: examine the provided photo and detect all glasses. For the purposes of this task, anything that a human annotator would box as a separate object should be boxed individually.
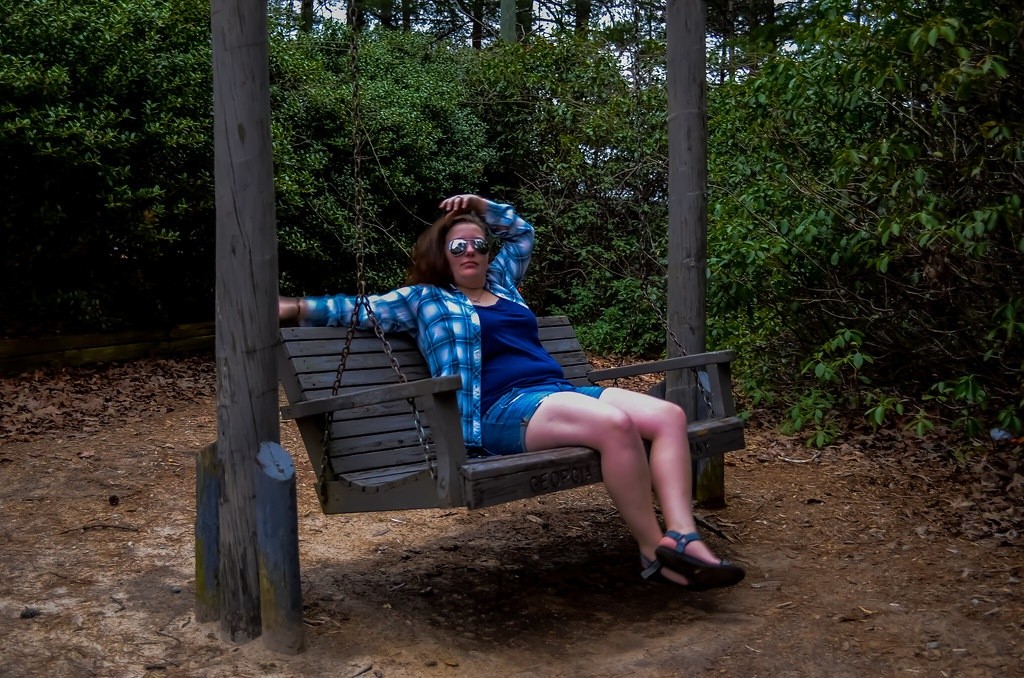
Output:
[444,237,490,255]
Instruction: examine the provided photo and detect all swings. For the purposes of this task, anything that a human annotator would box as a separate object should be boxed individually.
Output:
[272,0,748,515]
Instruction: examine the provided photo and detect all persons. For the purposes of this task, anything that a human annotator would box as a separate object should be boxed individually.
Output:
[278,194,748,593]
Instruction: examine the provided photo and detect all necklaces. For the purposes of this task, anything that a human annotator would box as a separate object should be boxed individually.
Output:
[459,288,484,305]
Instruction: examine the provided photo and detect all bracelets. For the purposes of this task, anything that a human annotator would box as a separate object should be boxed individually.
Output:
[296,297,302,321]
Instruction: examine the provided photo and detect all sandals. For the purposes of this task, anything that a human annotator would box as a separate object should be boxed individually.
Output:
[639,554,707,591]
[656,529,747,587]
